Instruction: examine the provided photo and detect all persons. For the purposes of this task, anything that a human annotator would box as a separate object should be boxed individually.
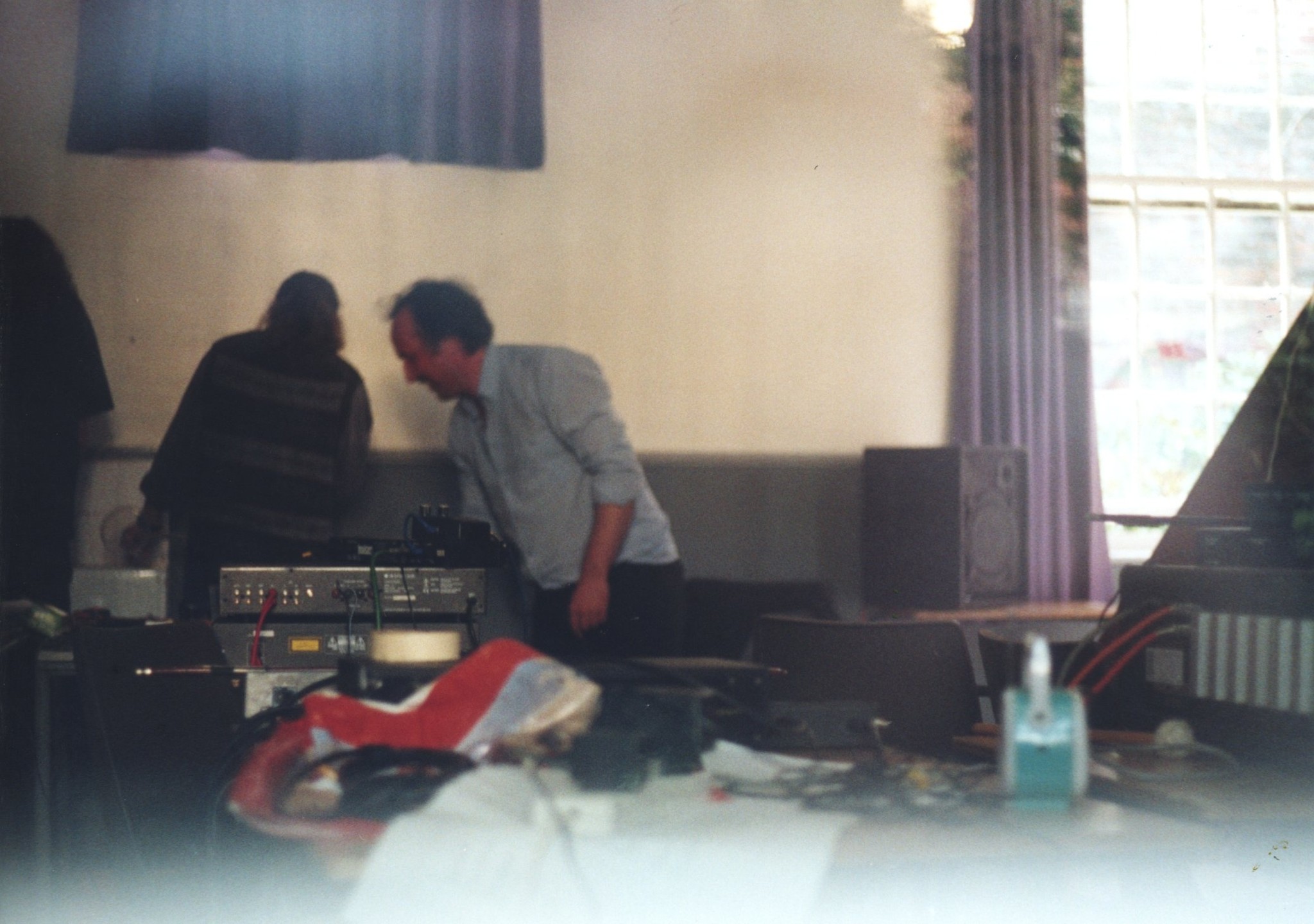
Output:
[122,270,378,664]
[388,279,677,788]
[0,213,117,725]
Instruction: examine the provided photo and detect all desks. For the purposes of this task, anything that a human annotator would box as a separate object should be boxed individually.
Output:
[764,600,1122,727]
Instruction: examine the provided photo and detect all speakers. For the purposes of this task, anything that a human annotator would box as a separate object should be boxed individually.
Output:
[861,445,1030,612]
